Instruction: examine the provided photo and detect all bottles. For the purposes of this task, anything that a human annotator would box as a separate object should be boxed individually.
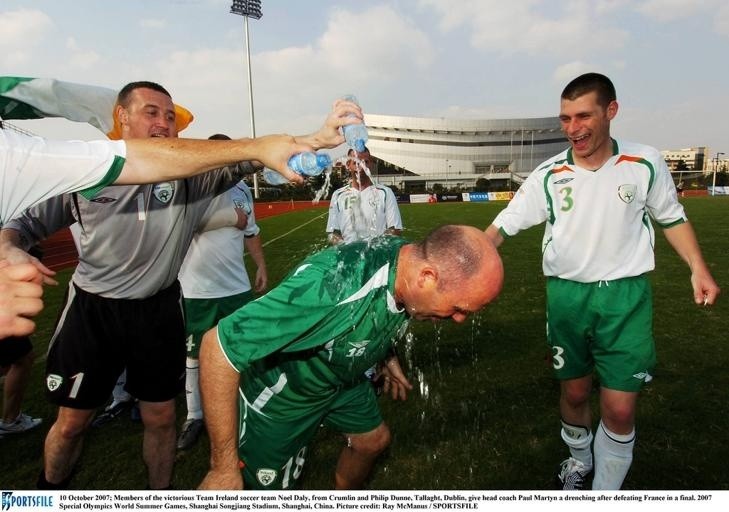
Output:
[340,94,368,154]
[263,153,331,186]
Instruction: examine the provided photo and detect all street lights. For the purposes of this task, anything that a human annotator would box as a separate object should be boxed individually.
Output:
[716,152,725,172]
[230,0,263,200]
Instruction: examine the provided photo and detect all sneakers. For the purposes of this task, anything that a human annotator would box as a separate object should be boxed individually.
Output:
[558,456,593,490]
[0,410,43,437]
[177,418,204,450]
[92,397,143,428]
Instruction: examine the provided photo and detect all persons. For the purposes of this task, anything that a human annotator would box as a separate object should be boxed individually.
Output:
[477,70,718,488]
[1,79,373,489]
[676,180,685,193]
[1,113,325,339]
[96,368,136,422]
[324,142,404,395]
[195,223,503,490]
[169,132,272,451]
[1,333,42,435]
